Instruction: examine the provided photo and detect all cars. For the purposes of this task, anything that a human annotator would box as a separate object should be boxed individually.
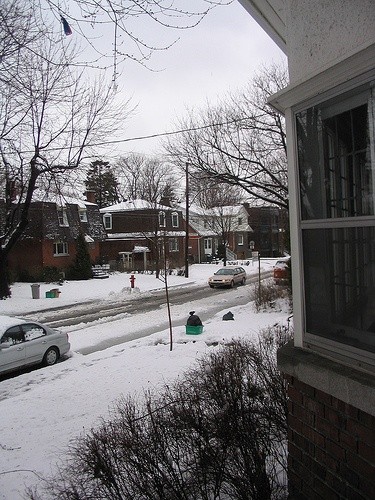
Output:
[0,316,71,375]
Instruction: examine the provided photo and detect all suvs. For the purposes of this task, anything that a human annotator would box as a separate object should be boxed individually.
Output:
[208,266,246,289]
[273,258,290,285]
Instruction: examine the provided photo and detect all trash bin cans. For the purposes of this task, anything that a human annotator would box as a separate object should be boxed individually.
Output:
[45,288,61,298]
[31,284,40,299]
[185,324,204,335]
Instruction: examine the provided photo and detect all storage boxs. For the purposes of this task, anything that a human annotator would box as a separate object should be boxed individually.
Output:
[185,324,204,335]
[46,289,61,298]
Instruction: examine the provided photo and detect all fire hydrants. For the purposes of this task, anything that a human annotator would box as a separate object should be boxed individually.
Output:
[129,275,137,288]
